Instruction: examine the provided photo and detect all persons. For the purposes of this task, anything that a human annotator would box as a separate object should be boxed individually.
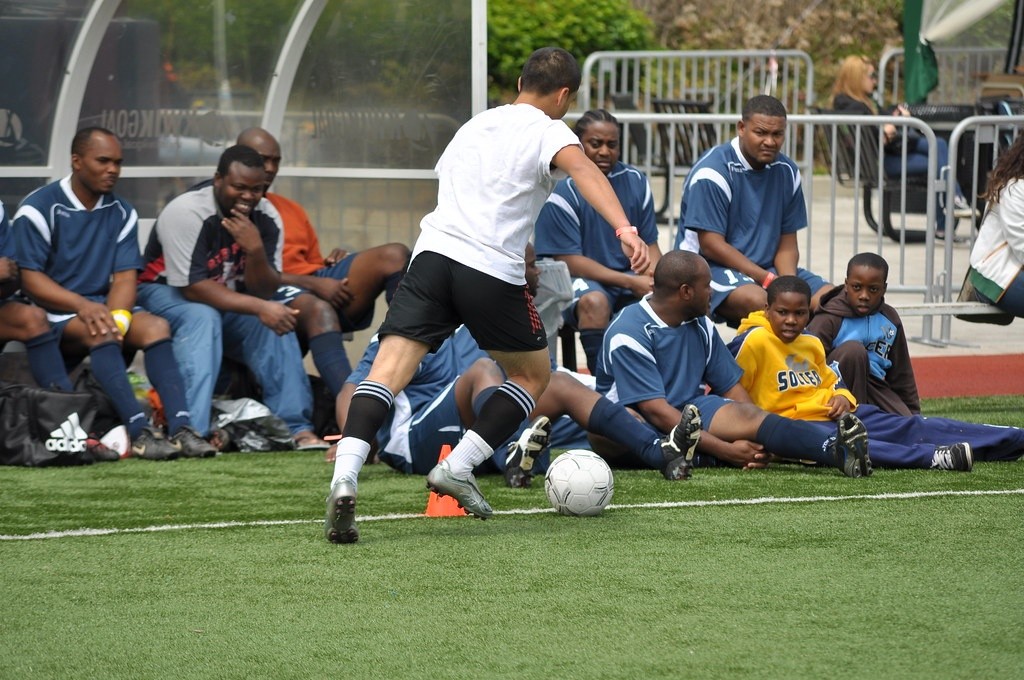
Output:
[969,130,1024,319]
[13,127,216,460]
[138,144,331,448]
[336,96,1024,488]
[828,54,981,248]
[237,127,410,395]
[324,47,651,544]
[0,199,120,461]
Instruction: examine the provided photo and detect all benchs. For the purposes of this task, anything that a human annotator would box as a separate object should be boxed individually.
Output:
[611,88,724,226]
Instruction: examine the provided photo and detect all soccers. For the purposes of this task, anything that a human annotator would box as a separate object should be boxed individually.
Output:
[544,449,614,516]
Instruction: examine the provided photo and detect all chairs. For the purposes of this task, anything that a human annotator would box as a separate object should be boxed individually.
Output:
[809,106,967,241]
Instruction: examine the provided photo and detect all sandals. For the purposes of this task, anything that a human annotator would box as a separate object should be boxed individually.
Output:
[291,432,332,450]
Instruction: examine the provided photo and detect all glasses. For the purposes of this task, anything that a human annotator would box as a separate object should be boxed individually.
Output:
[863,70,878,80]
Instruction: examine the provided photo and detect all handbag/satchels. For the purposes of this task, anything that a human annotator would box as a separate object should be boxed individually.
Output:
[0,364,116,468]
[953,266,1016,326]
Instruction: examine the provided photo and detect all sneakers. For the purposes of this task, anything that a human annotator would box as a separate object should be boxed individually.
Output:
[827,412,873,478]
[323,475,359,544]
[168,427,216,457]
[929,442,973,471]
[654,401,703,481]
[426,459,493,521]
[131,431,182,461]
[505,414,552,488]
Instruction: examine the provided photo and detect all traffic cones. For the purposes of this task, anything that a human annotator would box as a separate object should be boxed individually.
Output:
[426,444,467,517]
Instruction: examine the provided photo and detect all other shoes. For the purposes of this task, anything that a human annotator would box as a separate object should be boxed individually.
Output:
[934,230,973,249]
[944,194,981,218]
[206,429,228,449]
[85,434,122,461]
[53,447,98,465]
